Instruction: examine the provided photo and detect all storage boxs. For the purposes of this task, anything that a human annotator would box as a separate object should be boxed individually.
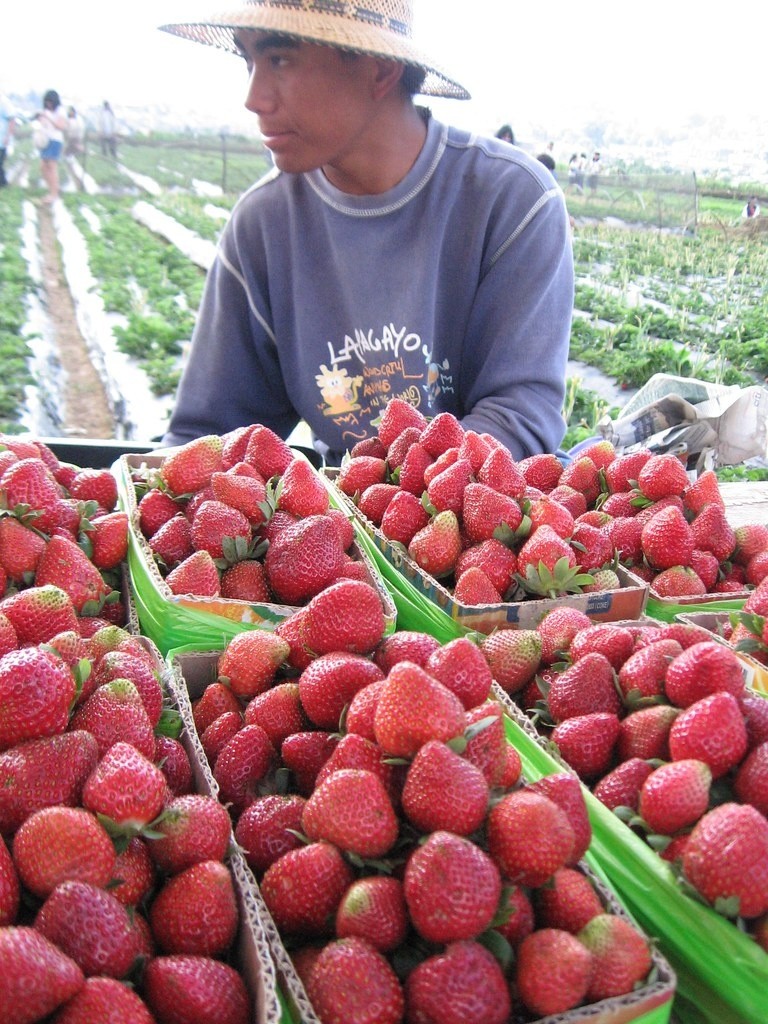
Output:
[13,436,768,1023]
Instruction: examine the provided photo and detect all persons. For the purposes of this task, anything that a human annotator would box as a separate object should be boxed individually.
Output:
[160,0,578,469]
[34,91,116,204]
[0,95,16,188]
[536,154,576,227]
[742,196,760,218]
[568,152,601,198]
[496,125,517,145]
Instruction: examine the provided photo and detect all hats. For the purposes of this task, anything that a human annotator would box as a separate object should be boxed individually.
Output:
[155,0,472,100]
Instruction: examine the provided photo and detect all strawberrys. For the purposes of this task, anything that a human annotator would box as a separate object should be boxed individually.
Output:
[0,396,768,1024]
